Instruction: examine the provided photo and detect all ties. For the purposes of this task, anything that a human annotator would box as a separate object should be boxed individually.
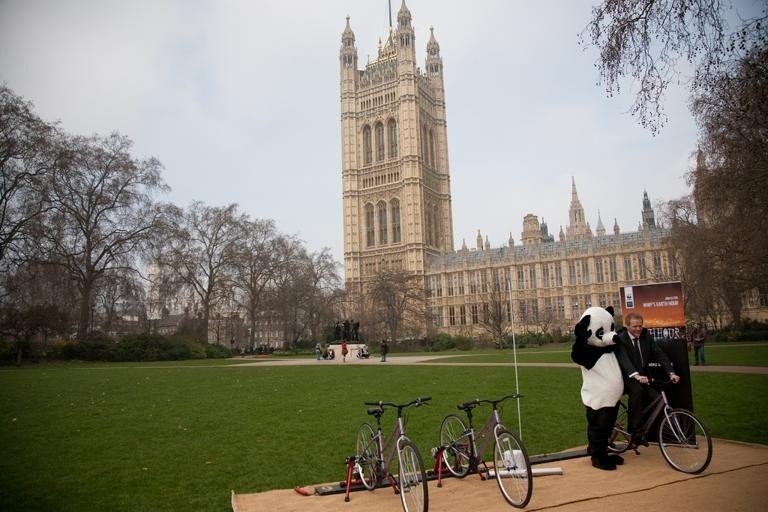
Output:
[633,338,643,368]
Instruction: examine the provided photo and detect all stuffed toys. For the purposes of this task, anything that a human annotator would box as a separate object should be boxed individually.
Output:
[570,305,625,471]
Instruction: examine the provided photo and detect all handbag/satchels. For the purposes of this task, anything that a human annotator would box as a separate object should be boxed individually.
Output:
[688,342,692,351]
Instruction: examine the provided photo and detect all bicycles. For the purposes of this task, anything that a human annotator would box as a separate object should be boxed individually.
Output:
[353,395,434,507]
[606,376,714,474]
[441,394,535,508]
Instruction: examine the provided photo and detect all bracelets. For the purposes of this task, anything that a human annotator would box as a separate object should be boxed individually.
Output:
[670,372,676,375]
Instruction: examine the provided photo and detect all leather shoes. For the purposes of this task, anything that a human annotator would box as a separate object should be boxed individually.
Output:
[627,424,644,434]
[631,435,647,445]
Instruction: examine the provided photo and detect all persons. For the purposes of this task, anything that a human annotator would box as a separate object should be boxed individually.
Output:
[341,340,349,363]
[241,345,245,358]
[380,339,388,362]
[613,311,680,447]
[316,343,321,360]
[357,346,370,359]
[334,319,360,341]
[689,322,707,366]
[322,343,335,360]
